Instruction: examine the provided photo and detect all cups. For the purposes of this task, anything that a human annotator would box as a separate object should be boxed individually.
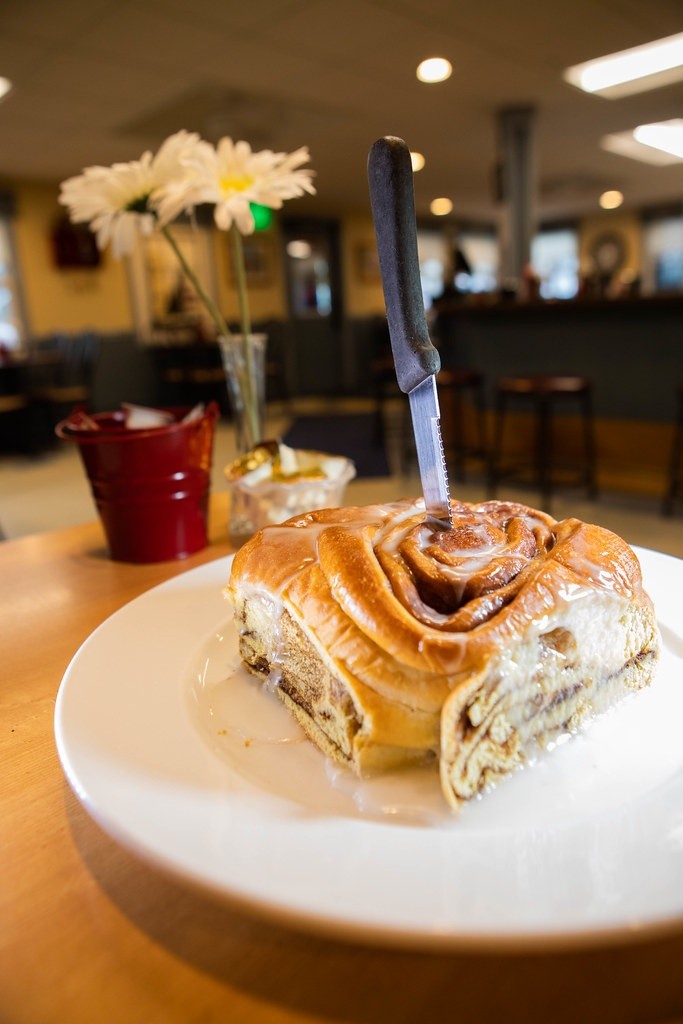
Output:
[55,410,220,565]
[225,456,356,548]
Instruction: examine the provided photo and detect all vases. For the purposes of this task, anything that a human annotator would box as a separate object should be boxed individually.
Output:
[217,333,266,535]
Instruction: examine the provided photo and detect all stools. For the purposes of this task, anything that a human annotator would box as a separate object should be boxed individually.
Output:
[372,368,476,477]
[486,374,599,514]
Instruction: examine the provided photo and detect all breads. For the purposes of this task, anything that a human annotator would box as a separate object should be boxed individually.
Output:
[227,499,663,807]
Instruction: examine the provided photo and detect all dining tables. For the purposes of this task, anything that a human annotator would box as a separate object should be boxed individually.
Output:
[0,478,683,1024]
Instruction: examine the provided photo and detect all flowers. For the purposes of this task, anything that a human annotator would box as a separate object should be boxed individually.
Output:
[61,129,317,504]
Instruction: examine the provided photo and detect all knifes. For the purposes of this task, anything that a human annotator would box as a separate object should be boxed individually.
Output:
[365,136,454,531]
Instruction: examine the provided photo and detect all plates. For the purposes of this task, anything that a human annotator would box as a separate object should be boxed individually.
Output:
[51,543,683,955]
